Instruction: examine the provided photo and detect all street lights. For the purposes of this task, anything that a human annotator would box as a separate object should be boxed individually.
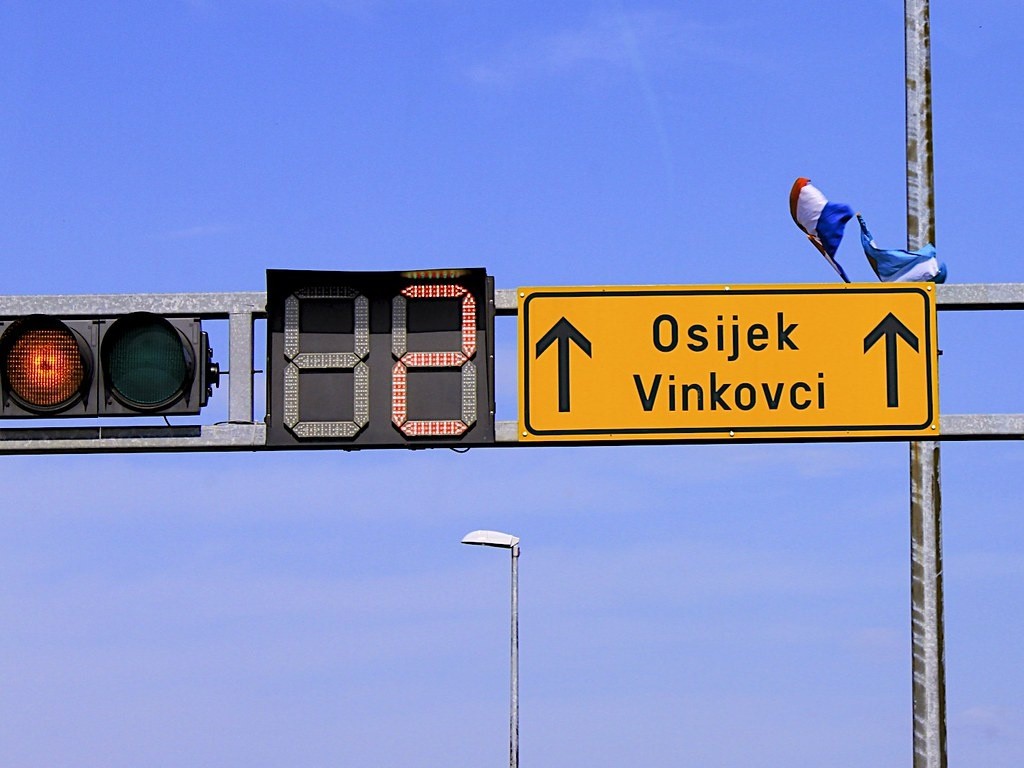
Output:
[459,528,526,768]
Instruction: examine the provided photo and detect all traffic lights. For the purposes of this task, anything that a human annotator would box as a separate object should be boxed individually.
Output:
[1,309,222,420]
[263,266,497,450]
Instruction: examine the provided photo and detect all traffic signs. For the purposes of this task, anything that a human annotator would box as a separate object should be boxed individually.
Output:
[517,282,942,445]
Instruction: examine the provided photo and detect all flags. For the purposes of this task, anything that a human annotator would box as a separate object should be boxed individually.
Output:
[857,215,948,284]
[789,178,855,283]
